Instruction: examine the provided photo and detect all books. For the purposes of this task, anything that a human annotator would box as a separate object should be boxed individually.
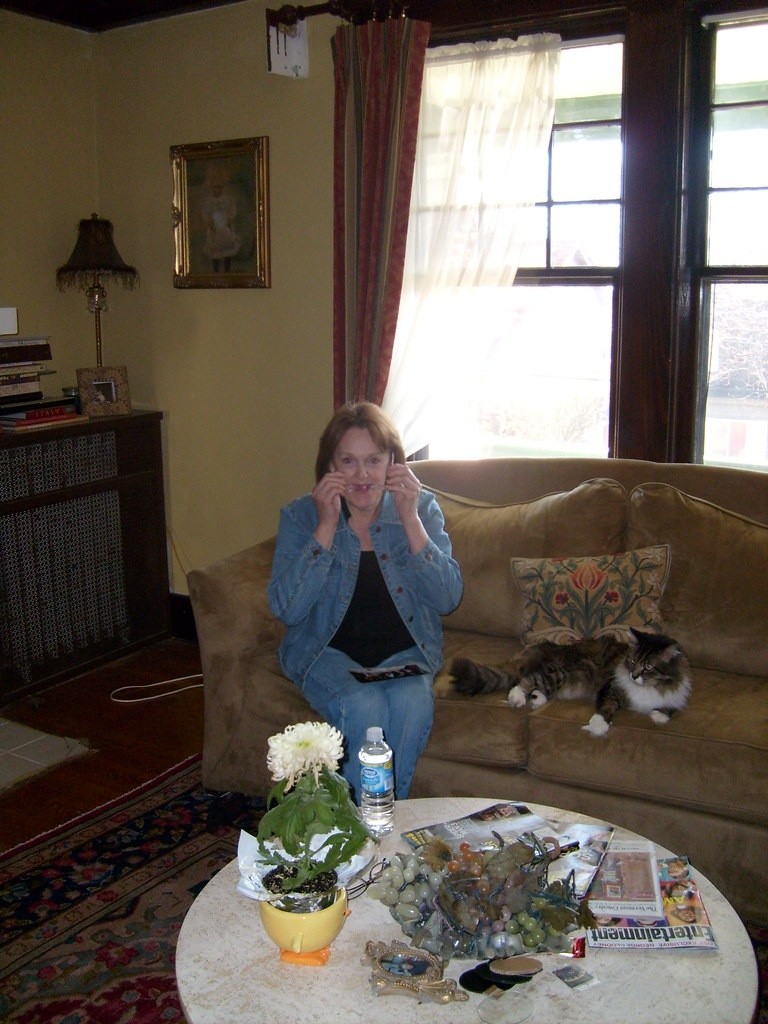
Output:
[400,800,719,952]
[0,335,89,431]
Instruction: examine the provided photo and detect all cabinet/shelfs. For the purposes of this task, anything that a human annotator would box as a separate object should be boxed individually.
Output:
[0,406,177,706]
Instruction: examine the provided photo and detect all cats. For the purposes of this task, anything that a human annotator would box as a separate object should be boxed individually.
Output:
[446,626,694,737]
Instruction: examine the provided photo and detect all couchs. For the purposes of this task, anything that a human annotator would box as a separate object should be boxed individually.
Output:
[186,457,767,939]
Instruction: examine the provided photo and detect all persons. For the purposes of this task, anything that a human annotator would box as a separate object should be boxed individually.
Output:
[398,960,433,976]
[200,176,239,272]
[268,402,464,807]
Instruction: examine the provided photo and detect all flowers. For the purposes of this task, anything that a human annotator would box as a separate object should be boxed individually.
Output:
[257,720,371,889]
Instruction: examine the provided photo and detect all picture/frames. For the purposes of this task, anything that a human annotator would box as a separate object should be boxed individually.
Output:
[77,366,132,417]
[169,136,274,290]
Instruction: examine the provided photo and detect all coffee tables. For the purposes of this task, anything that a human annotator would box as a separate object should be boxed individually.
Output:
[174,797,757,1023]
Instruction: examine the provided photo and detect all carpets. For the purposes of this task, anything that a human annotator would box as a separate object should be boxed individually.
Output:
[0,748,768,1024]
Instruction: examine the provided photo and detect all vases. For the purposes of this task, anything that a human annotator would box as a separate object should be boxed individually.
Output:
[258,886,351,967]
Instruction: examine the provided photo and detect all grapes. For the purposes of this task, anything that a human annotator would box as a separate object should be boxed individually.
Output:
[366,843,563,948]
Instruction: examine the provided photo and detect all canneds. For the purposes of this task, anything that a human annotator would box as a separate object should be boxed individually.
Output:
[62,387,83,415]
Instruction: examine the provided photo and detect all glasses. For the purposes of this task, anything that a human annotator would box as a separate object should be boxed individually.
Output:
[335,452,395,493]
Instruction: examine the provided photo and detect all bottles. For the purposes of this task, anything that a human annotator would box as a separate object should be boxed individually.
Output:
[358,727,395,837]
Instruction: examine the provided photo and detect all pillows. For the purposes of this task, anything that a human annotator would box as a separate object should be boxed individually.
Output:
[510,543,672,648]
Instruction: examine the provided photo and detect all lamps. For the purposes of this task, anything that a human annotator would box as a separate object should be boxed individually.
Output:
[55,212,142,367]
